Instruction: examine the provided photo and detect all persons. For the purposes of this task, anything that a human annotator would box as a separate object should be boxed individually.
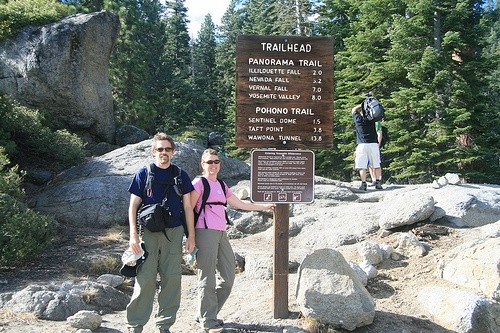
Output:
[353,105,384,190]
[190,149,276,333]
[126,133,194,333]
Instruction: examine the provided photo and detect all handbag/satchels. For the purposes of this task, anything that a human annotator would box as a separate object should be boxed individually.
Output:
[137,204,167,232]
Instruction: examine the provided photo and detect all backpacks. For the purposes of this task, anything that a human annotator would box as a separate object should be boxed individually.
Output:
[182,177,227,238]
[362,97,384,122]
[380,126,389,148]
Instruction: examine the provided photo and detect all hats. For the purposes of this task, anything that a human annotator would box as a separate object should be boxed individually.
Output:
[119,242,148,277]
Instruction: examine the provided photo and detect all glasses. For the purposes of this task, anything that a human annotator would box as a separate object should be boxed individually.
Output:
[203,160,220,164]
[155,147,173,152]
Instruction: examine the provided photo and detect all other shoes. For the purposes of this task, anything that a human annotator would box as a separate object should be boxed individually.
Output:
[199,323,224,333]
[375,181,383,189]
[368,181,376,187]
[196,316,224,325]
[359,183,367,191]
